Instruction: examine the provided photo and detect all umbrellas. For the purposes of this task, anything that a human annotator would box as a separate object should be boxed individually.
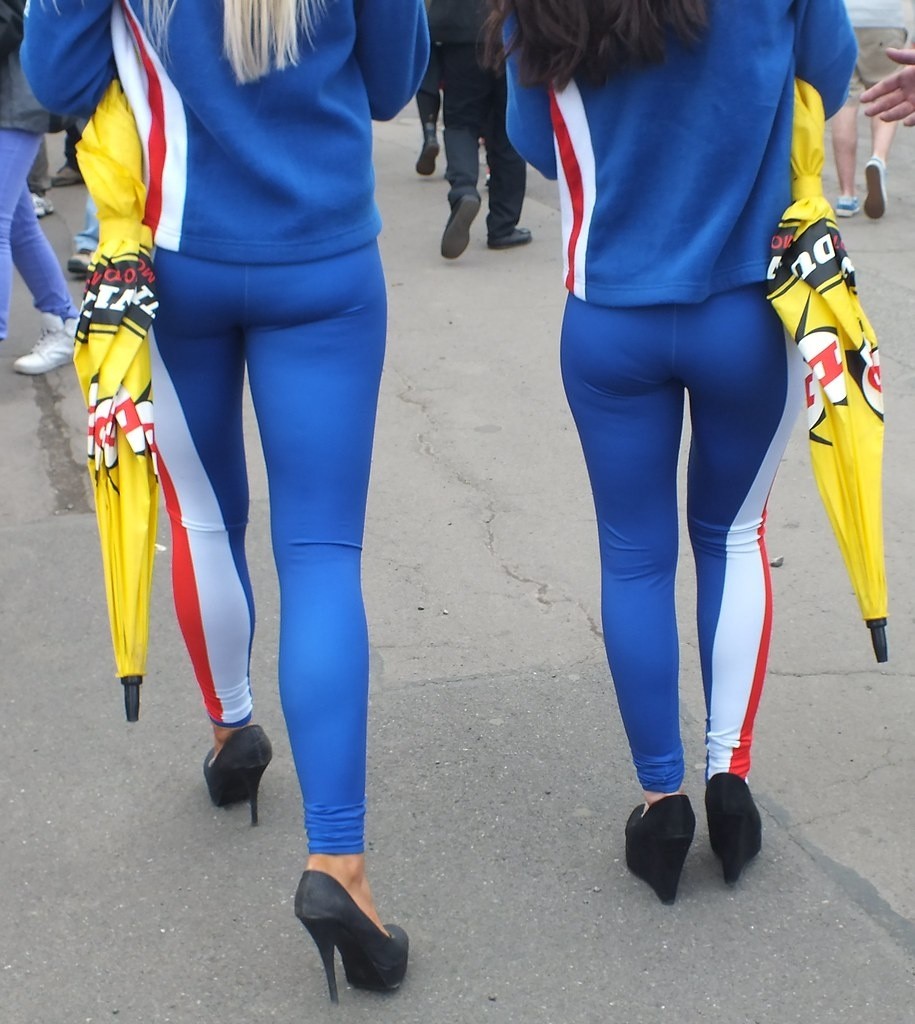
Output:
[764,74,893,665]
[71,74,159,727]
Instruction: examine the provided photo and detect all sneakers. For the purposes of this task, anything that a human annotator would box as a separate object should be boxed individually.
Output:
[415,136,440,177]
[30,193,53,219]
[865,156,888,221]
[834,195,862,218]
[66,247,96,274]
[11,312,83,376]
[50,164,83,185]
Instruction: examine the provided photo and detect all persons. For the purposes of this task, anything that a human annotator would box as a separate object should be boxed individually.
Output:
[15,0,428,1005]
[437,0,535,260]
[406,0,459,179]
[0,0,99,376]
[822,1,907,221]
[857,37,914,131]
[474,1,863,910]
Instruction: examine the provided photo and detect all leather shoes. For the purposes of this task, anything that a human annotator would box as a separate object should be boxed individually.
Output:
[485,226,531,249]
[439,191,481,260]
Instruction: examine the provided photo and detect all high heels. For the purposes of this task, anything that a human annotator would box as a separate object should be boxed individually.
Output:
[293,870,410,1008]
[202,723,274,829]
[624,792,697,907]
[704,772,762,889]
